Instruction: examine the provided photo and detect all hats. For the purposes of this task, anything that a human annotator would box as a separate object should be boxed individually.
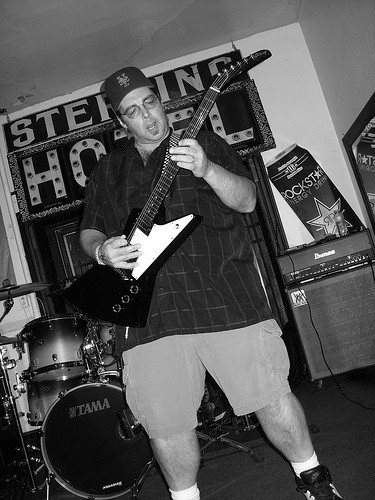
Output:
[105,66,154,111]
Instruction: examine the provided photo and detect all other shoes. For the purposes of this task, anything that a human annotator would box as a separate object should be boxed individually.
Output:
[295,466,344,500]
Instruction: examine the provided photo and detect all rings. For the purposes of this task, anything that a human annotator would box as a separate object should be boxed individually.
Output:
[192,156,195,163]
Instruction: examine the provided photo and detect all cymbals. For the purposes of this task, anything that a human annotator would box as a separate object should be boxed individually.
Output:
[0,280,52,302]
[0,334,20,346]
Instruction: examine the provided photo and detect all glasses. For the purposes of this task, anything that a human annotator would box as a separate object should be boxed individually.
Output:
[121,95,160,116]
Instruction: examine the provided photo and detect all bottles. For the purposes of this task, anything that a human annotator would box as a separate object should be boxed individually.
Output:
[333,210,347,236]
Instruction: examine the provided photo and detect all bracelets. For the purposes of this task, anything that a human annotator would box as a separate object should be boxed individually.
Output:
[96,245,105,265]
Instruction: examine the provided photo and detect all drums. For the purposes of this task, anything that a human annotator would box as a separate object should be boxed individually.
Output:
[27,364,103,427]
[92,321,122,380]
[39,381,155,500]
[21,311,98,383]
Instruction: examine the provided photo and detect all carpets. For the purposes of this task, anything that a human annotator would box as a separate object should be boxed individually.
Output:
[196,413,322,461]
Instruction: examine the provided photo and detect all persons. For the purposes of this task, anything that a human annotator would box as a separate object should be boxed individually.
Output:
[79,66,343,500]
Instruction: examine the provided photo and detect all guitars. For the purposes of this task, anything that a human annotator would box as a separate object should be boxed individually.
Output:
[44,48,274,329]
